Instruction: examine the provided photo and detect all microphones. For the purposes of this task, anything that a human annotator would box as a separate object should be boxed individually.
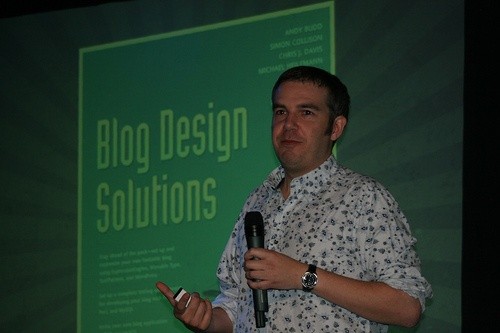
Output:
[243,211,269,328]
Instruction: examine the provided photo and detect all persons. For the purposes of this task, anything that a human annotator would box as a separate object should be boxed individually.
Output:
[155,66,432,332]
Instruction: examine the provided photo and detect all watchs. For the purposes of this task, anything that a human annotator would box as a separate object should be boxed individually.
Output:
[301,263,319,293]
